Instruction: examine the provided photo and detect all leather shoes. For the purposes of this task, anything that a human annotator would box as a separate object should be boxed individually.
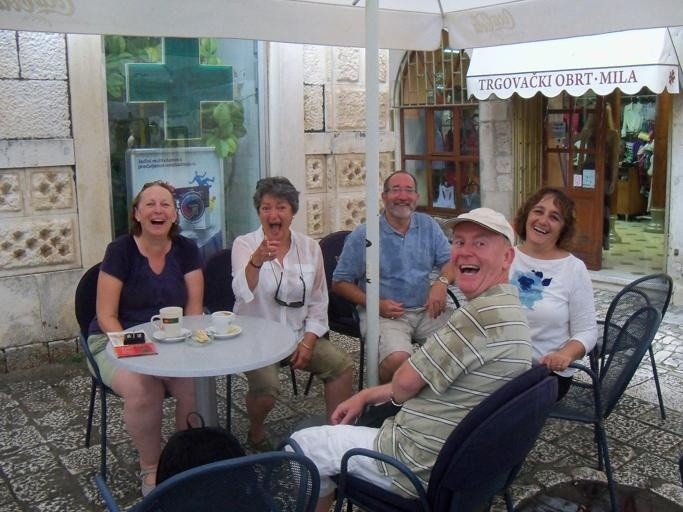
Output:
[139,469,157,498]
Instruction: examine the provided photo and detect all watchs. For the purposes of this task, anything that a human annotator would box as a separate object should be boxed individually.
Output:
[438,276,449,286]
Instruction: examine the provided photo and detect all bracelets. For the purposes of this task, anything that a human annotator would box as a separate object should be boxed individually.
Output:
[389,391,402,406]
[249,260,263,269]
[301,342,313,350]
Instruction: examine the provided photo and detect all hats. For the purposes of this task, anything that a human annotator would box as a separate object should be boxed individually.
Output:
[443,206,514,250]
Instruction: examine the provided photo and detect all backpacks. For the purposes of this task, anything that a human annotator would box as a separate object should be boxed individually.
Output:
[155,412,259,511]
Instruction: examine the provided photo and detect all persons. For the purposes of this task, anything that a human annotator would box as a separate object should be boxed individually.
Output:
[85,181,206,497]
[509,186,599,402]
[284,208,532,511]
[581,102,620,242]
[230,176,354,452]
[331,170,456,384]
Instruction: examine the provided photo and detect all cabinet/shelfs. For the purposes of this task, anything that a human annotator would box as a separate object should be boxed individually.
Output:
[616,164,643,222]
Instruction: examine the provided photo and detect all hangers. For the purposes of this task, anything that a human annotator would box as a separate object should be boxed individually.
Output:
[629,95,657,107]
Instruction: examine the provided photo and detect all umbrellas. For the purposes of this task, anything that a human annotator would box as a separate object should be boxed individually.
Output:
[0,0,683,388]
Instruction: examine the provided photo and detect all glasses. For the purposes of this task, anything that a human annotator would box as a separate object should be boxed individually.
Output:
[384,188,416,194]
[274,271,306,309]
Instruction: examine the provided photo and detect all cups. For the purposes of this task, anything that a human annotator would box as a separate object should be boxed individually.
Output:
[151,307,183,338]
[212,311,235,334]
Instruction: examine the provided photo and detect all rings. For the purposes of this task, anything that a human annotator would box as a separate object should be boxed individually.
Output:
[268,252,271,256]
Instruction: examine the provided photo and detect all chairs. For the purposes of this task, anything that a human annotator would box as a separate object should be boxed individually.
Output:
[303,230,460,398]
[548,307,663,511]
[93,439,322,512]
[74,260,199,482]
[582,273,676,444]
[201,249,299,435]
[329,364,560,512]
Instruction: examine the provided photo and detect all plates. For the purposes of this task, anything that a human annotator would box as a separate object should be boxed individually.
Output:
[205,325,242,339]
[153,328,192,344]
